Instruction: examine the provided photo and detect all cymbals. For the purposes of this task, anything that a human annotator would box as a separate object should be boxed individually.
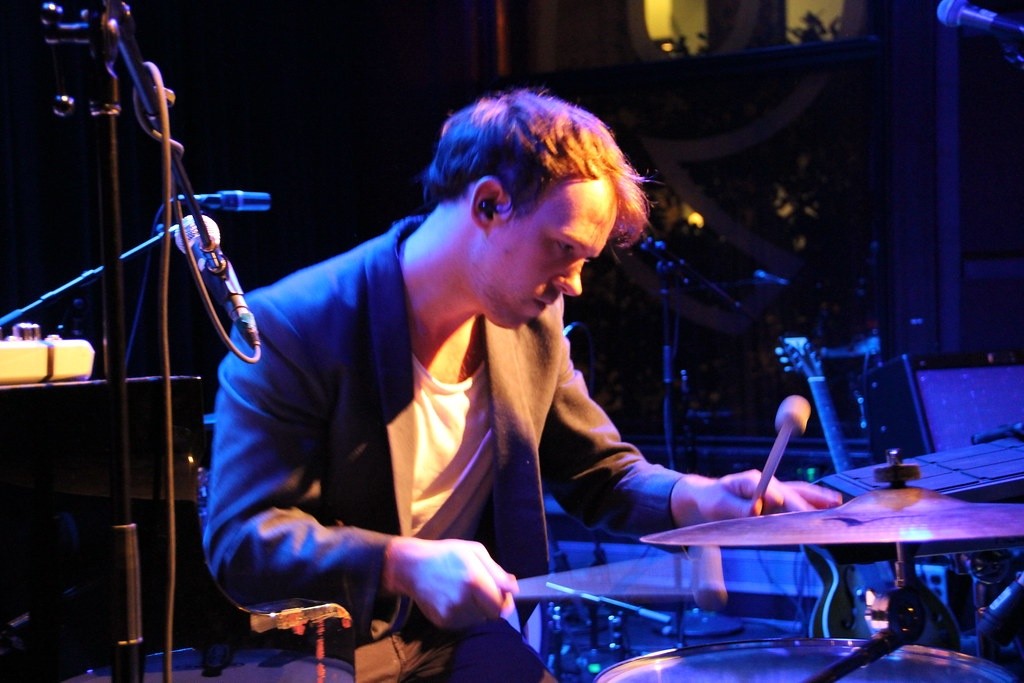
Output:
[640,486,1024,546]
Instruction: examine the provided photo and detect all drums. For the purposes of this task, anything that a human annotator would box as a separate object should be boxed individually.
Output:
[592,639,1019,683]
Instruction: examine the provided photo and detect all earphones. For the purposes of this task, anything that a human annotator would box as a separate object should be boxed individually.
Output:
[480,198,500,220]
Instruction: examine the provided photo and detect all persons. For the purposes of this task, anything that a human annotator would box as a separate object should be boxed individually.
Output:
[202,92,842,683]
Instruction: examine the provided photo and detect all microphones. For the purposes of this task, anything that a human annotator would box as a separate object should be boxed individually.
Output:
[176,214,262,348]
[756,271,789,285]
[190,191,271,215]
[980,574,1024,649]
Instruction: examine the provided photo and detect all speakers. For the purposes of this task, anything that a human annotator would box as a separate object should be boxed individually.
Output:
[860,346,1024,464]
